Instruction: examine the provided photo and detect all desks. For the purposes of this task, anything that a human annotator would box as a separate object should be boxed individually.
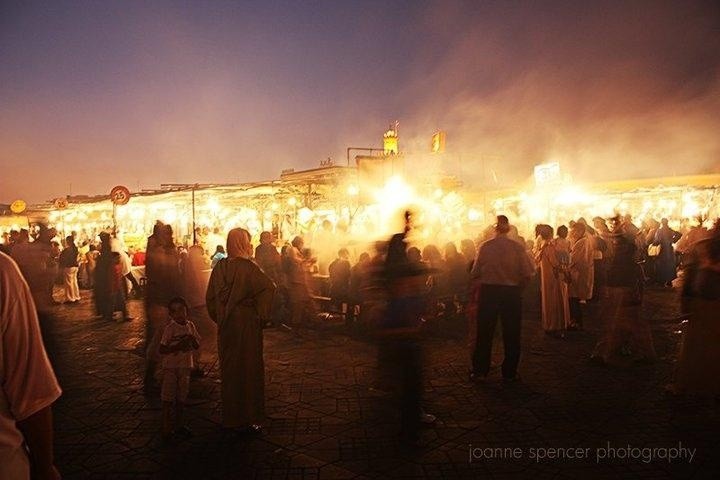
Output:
[121,263,333,318]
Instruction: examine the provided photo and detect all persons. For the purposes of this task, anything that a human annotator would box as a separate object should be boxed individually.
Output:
[1,250,63,479]
[0,214,720,427]
